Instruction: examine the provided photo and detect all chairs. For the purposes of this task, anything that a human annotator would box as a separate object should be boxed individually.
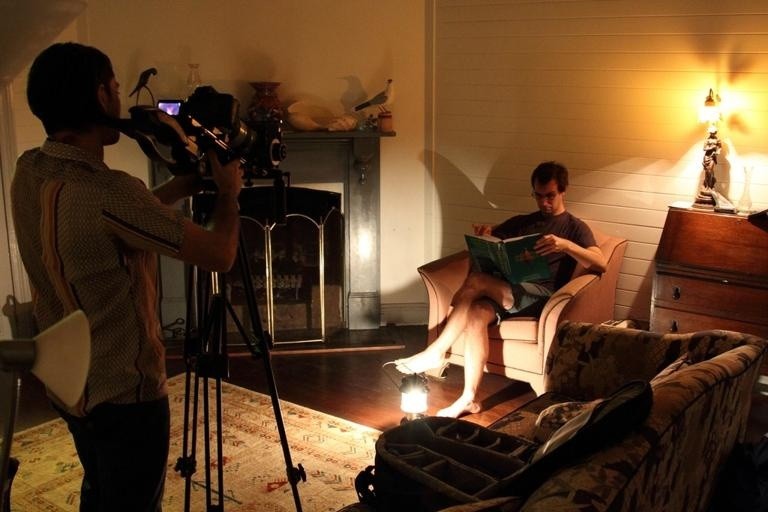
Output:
[412,234,630,400]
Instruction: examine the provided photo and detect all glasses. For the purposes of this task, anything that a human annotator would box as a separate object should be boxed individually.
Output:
[527,187,557,200]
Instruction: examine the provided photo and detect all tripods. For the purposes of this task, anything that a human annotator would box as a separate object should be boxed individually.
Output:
[172,192,307,512]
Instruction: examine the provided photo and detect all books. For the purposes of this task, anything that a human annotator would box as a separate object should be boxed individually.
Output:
[463,232,552,285]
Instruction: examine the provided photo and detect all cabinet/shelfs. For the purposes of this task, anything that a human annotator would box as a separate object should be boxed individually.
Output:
[641,201,767,405]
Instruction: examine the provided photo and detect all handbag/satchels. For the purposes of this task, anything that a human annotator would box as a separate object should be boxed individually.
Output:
[353,379,652,509]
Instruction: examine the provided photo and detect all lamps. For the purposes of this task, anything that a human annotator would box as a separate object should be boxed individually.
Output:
[702,81,724,124]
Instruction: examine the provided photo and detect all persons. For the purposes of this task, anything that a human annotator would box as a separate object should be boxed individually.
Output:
[698,126,724,196]
[8,39,245,512]
[395,161,609,421]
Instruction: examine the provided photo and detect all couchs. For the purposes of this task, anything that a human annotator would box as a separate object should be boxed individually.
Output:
[367,317,767,512]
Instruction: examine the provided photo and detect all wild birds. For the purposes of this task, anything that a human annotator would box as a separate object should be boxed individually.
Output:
[354,77,394,113]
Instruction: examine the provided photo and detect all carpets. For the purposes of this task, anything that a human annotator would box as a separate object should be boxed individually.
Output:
[0,372,379,512]
